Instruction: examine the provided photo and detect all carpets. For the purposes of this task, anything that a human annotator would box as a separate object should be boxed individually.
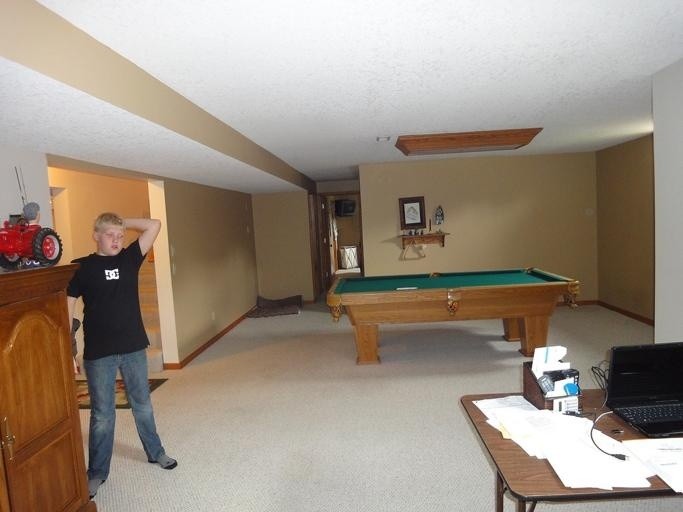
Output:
[244,295,303,317]
[72,376,168,408]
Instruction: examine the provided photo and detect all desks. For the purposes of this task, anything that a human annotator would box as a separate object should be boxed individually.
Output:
[460,387,682,512]
[326,267,580,366]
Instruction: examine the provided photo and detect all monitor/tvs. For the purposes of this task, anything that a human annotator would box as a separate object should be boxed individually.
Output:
[335,199,356,215]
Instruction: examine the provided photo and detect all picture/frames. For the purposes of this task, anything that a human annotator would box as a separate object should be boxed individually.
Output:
[398,196,425,230]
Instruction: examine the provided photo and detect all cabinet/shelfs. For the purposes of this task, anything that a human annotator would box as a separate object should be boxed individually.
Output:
[0,262,97,511]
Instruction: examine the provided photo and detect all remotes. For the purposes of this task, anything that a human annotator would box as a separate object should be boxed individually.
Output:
[537,374,554,395]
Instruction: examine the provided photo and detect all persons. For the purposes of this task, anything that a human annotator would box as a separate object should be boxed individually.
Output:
[66,212,177,500]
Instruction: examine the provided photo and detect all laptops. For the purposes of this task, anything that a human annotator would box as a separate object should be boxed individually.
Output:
[606,342,683,438]
